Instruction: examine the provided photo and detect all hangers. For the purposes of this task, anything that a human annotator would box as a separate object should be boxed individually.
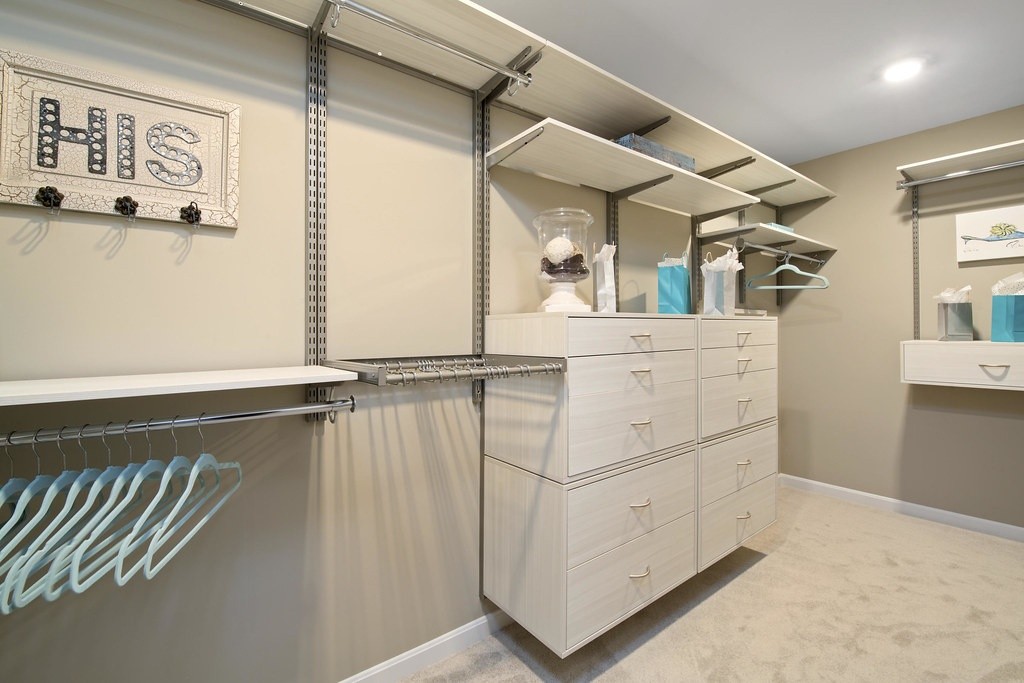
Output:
[741,254,829,288]
[0,410,244,615]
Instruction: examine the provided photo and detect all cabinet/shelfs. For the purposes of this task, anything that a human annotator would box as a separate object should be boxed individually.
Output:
[698,420,778,572]
[900,339,1024,390]
[483,444,698,657]
[698,314,778,443]
[486,312,698,484]
[243,0,838,257]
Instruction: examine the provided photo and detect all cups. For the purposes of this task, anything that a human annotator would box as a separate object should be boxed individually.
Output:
[532,207,595,283]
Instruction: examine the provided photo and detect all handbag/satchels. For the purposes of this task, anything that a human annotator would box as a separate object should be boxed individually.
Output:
[703,249,738,319]
[991,294,1024,342]
[658,251,692,314]
[593,240,618,313]
[937,292,973,341]
